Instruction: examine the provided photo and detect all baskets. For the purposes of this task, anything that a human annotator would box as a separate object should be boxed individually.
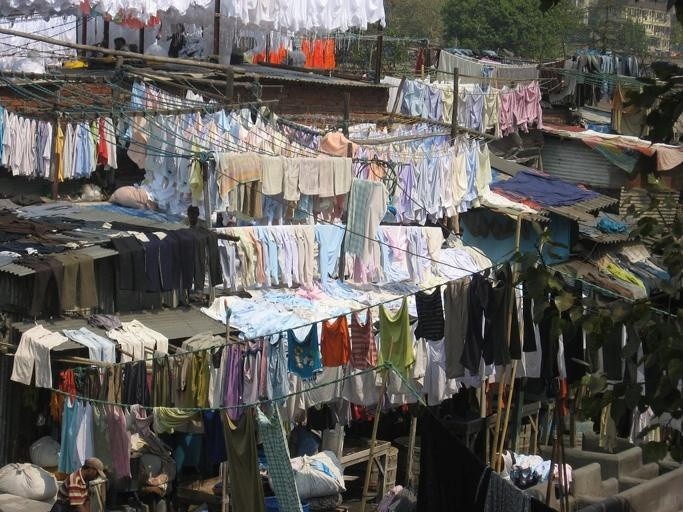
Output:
[263,495,310,512]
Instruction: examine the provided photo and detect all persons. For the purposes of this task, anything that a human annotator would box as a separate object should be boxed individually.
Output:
[114,37,135,53]
[57,457,108,512]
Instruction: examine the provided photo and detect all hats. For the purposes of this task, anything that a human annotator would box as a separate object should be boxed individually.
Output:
[85,457,107,479]
